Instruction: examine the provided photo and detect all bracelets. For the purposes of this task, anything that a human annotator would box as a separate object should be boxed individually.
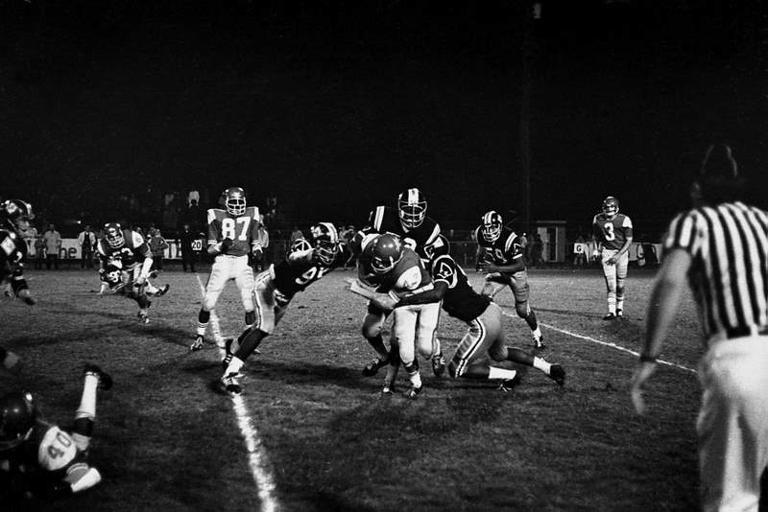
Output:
[639,350,660,366]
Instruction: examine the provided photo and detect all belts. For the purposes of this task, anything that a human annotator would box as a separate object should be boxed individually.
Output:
[725,326,768,338]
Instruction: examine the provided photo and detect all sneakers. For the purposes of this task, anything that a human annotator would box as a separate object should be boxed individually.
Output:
[362,350,393,377]
[222,339,236,370]
[190,333,206,352]
[546,363,566,387]
[83,363,113,391]
[400,378,424,400]
[494,369,521,394]
[138,308,151,324]
[154,283,170,298]
[381,377,396,396]
[431,349,446,376]
[218,375,242,398]
[602,309,625,320]
[532,330,545,350]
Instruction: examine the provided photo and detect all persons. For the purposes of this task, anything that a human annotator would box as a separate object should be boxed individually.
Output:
[625,133,767,512]
[0,362,116,511]
[0,197,37,373]
[590,192,634,322]
[572,234,586,266]
[40,222,63,269]
[34,236,45,270]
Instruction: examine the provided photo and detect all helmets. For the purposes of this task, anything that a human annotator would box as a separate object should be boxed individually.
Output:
[397,187,428,230]
[480,210,504,243]
[368,233,404,275]
[1,392,40,452]
[310,221,340,266]
[602,196,620,217]
[224,187,247,217]
[1,199,33,238]
[438,232,452,256]
[104,222,126,249]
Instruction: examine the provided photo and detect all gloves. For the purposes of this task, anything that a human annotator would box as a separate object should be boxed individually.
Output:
[251,244,263,263]
[214,237,234,253]
